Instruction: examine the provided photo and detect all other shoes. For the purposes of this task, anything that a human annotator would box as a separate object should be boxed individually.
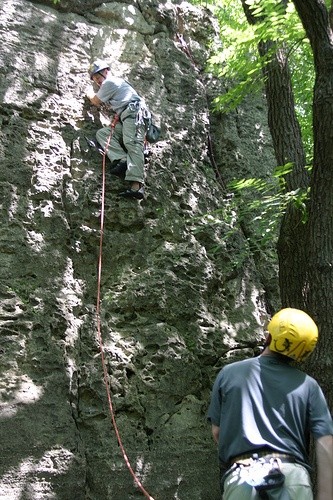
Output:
[110,160,127,172]
[119,185,144,199]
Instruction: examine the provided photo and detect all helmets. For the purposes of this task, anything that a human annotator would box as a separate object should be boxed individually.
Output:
[88,60,110,80]
[267,308,318,364]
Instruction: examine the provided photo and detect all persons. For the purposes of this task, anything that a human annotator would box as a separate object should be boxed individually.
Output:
[85,59,148,199]
[208,308,333,500]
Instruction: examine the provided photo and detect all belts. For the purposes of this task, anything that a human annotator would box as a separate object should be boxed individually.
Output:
[231,451,290,464]
